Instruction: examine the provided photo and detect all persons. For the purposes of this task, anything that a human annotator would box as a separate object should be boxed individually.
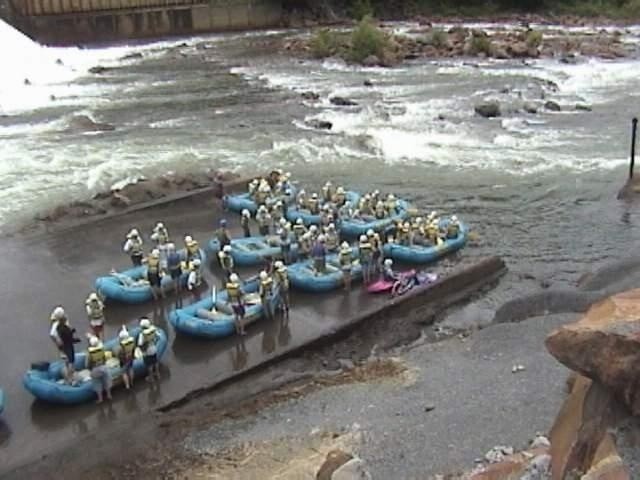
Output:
[85,291,105,344]
[137,318,161,382]
[186,258,204,304]
[140,248,167,301]
[151,221,169,251]
[183,235,203,262]
[117,326,137,391]
[84,332,114,404]
[123,227,143,267]
[167,242,184,296]
[50,305,81,384]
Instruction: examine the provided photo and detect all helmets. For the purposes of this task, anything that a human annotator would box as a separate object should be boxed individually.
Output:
[259,271,269,281]
[238,171,459,267]
[51,306,65,320]
[86,293,151,346]
[125,218,234,268]
[229,272,238,283]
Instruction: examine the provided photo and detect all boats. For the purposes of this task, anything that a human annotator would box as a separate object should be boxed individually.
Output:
[286,190,361,227]
[169,275,283,340]
[281,246,384,294]
[94,247,207,305]
[382,219,465,264]
[336,198,410,237]
[25,325,167,404]
[224,182,297,217]
[227,231,301,268]
